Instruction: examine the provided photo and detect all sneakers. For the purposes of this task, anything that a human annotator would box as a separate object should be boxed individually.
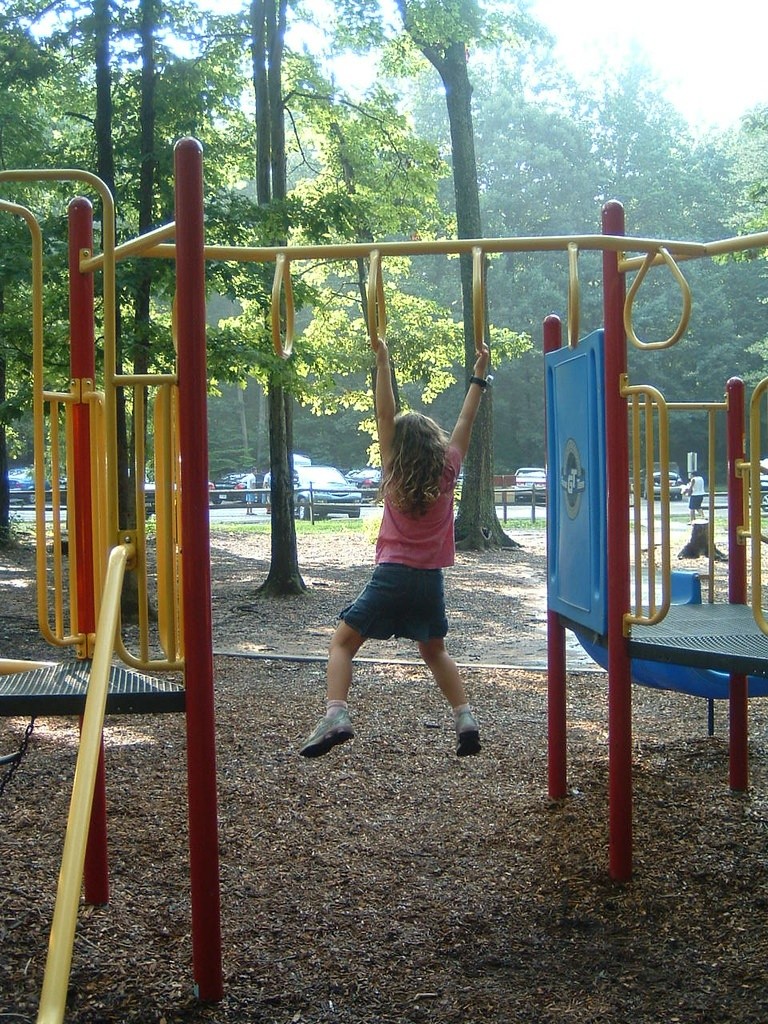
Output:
[299,710,356,758]
[455,710,481,756]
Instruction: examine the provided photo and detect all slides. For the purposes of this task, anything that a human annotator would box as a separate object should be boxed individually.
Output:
[564,573,767,701]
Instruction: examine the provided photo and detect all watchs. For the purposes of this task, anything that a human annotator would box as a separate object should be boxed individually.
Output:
[469,375,493,394]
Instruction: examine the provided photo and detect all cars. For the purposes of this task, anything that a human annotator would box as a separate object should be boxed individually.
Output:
[128,467,267,507]
[8,467,67,505]
[292,465,362,520]
[512,467,547,505]
[345,464,383,505]
[628,466,684,501]
[758,457,768,518]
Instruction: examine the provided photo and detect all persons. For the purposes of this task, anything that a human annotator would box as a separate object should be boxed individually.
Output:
[299,338,489,757]
[242,467,258,515]
[687,471,708,525]
[262,468,271,514]
